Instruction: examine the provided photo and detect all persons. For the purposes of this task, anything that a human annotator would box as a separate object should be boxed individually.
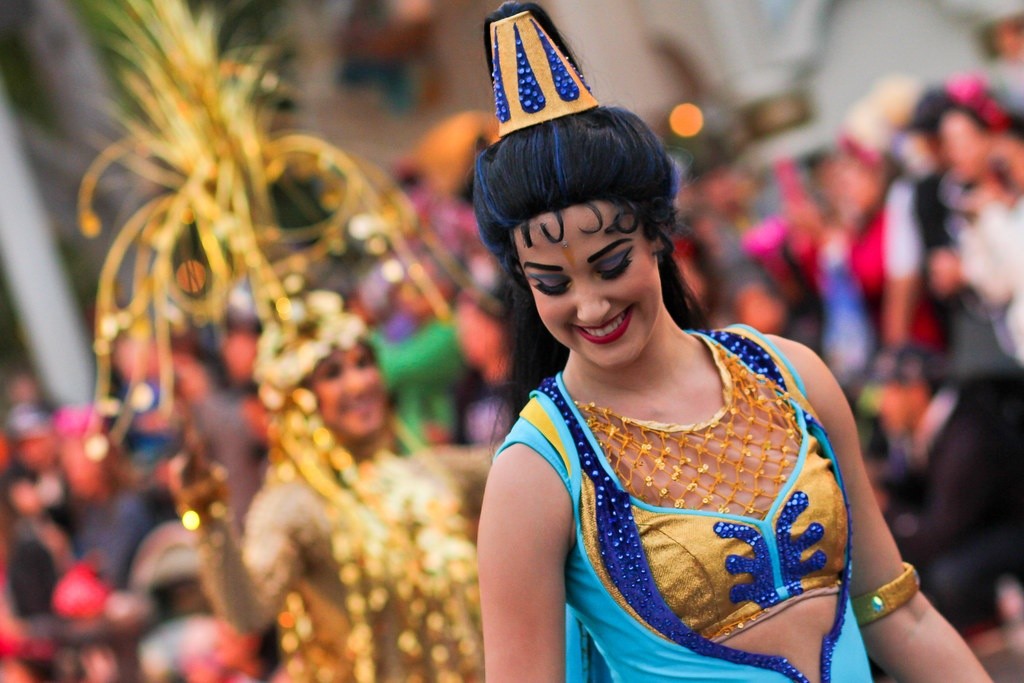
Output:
[473,101,1008,683]
[0,152,514,683]
[725,73,1022,682]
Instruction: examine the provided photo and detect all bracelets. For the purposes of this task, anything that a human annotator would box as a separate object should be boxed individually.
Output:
[168,454,230,529]
